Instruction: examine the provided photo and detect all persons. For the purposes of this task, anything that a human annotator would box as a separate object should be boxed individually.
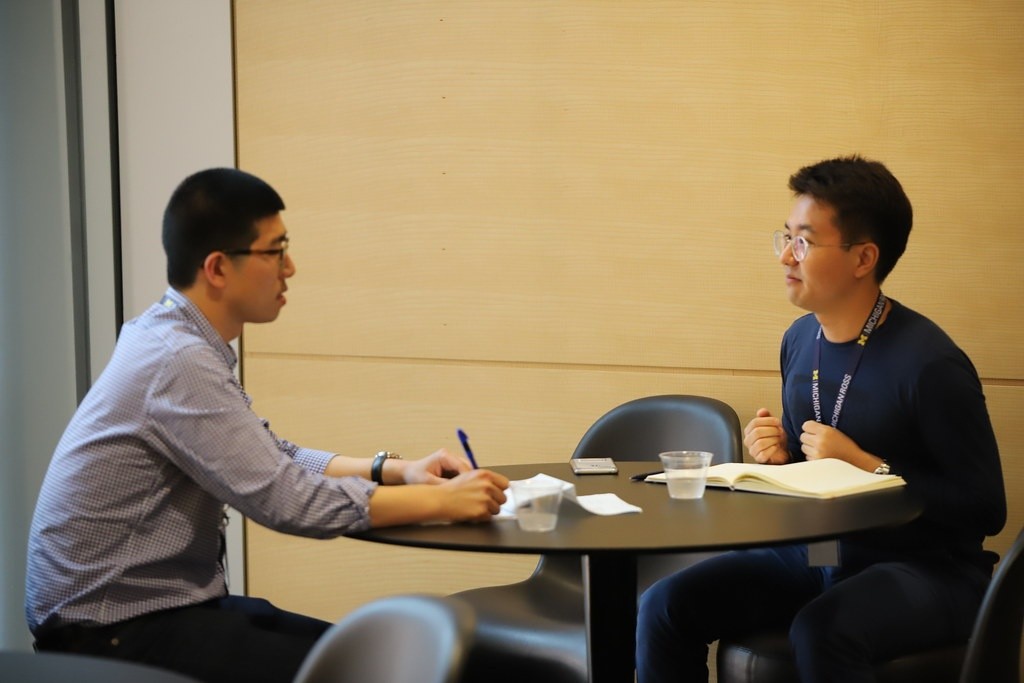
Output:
[25,169,509,683]
[636,156,1008,683]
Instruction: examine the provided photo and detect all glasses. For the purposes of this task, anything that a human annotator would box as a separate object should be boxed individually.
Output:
[772,230,866,261]
[201,237,288,269]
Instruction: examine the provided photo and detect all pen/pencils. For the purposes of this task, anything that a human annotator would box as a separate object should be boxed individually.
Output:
[457,428,479,469]
[628,470,664,479]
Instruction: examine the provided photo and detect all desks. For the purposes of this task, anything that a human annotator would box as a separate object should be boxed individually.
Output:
[342,461,924,683]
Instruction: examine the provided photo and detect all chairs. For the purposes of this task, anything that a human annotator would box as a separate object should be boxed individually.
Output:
[444,394,744,683]
[716,525,1024,683]
[0,646,197,683]
[293,593,475,683]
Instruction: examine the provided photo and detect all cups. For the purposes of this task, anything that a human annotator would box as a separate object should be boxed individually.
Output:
[658,450,714,499]
[511,481,564,532]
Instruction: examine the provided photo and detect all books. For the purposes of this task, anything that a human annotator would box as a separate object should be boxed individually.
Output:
[644,458,906,499]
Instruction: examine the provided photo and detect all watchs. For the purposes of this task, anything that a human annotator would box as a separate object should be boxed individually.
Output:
[372,451,402,485]
[875,459,890,474]
